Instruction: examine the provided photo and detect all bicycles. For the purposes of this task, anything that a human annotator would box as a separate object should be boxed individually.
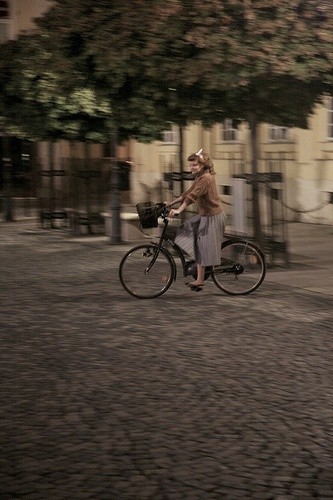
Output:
[118,199,267,301]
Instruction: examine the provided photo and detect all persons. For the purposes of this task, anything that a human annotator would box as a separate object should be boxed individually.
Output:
[163,149,226,287]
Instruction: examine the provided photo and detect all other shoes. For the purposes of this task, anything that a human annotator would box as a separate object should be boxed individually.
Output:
[185,282,205,287]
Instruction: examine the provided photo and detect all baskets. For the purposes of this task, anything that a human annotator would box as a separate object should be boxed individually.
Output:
[136,201,158,228]
